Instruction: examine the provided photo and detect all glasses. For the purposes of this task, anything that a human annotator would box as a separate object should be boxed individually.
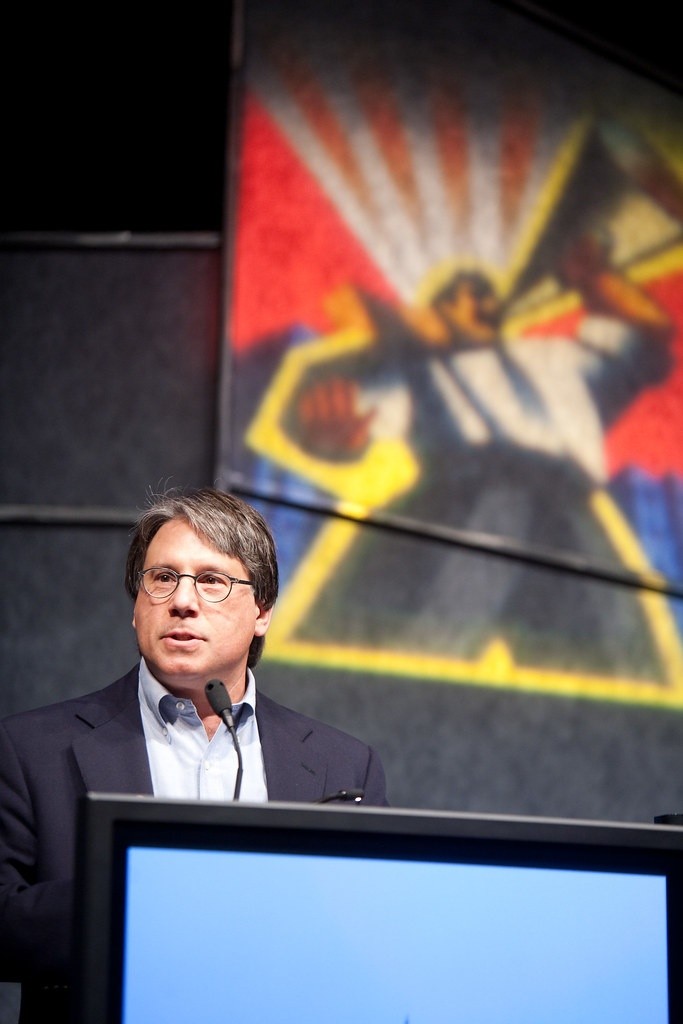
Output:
[136,567,252,602]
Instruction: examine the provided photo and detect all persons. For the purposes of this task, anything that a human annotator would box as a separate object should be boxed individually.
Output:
[0,487,392,1024]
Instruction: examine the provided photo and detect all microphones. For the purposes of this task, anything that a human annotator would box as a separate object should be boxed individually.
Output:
[203,678,243,801]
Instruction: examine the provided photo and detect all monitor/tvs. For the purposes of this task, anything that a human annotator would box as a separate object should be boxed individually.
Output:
[72,790,683,1024]
